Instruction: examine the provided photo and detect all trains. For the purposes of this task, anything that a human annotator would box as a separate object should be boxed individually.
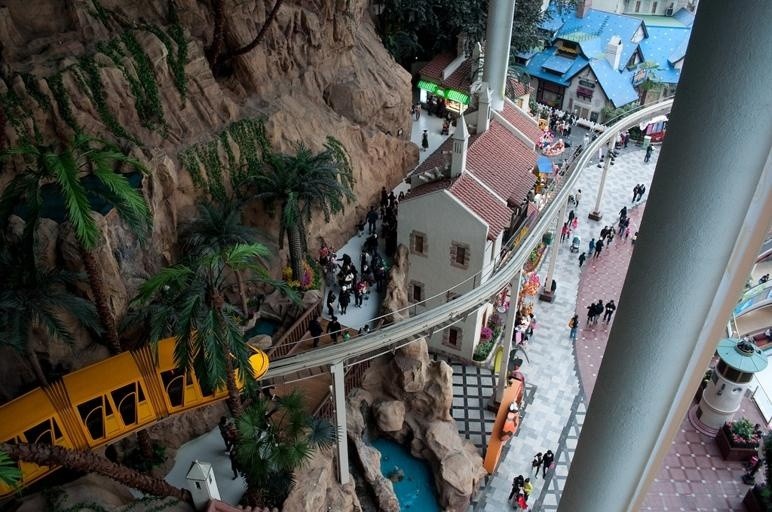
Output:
[0,329,269,495]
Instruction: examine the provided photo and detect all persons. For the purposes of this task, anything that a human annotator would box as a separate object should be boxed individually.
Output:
[217,414,248,481]
[568,298,616,339]
[631,183,646,204]
[506,449,554,512]
[309,186,411,348]
[624,131,631,148]
[421,97,455,152]
[515,310,537,337]
[644,145,654,162]
[559,189,638,268]
[529,100,579,140]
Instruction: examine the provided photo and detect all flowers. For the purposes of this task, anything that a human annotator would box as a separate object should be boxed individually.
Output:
[480,327,493,340]
[723,420,760,448]
